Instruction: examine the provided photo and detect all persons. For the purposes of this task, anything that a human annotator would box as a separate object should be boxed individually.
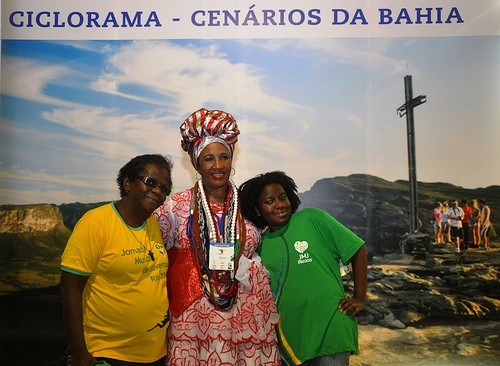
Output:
[480,199,492,250]
[60,154,174,366]
[432,200,452,245]
[460,200,473,249]
[151,108,281,366]
[446,200,465,253]
[237,170,367,366]
[470,199,481,248]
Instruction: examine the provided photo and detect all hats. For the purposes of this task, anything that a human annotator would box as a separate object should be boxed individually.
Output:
[179,108,240,170]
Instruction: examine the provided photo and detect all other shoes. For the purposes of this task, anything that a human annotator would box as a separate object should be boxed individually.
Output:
[460,244,490,250]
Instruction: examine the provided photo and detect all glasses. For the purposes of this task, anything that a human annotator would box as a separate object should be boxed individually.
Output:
[452,204,456,205]
[135,175,173,198]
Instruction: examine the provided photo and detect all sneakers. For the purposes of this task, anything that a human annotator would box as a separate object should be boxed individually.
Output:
[455,248,460,253]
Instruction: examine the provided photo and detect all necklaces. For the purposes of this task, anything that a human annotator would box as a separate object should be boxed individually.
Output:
[187,177,246,312]
[126,217,155,262]
